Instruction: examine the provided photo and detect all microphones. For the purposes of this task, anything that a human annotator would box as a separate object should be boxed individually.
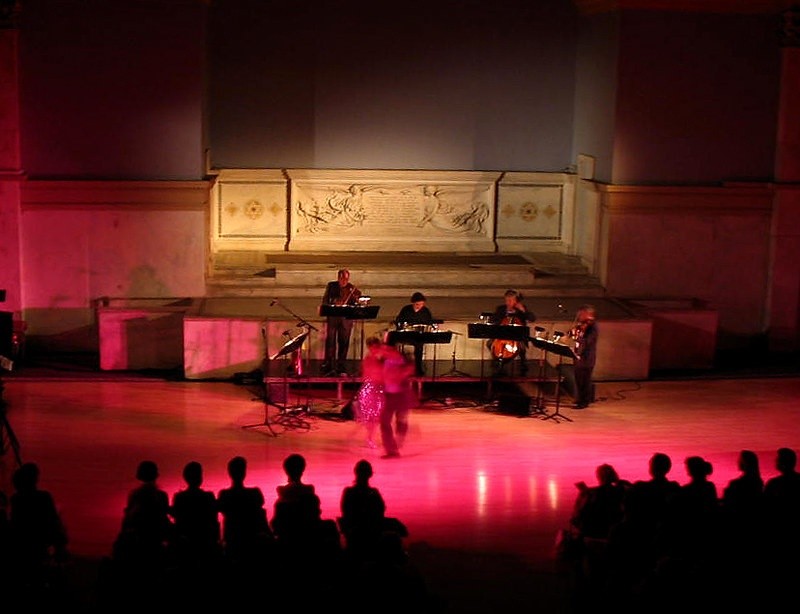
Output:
[270,297,277,306]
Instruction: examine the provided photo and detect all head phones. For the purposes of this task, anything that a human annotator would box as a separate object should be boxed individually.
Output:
[511,289,521,303]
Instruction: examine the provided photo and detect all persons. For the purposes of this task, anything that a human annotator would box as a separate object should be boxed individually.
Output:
[319,268,596,459]
[0,454,405,614]
[560,445,800,614]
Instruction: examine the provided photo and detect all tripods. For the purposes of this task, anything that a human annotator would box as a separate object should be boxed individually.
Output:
[242,332,310,439]
[440,331,470,377]
[532,340,576,422]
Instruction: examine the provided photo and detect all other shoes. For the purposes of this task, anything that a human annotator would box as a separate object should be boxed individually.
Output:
[571,400,589,409]
[337,363,352,372]
[325,368,338,377]
[396,433,405,449]
[380,451,401,459]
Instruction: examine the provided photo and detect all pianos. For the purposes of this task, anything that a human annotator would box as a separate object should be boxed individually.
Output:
[386,322,452,375]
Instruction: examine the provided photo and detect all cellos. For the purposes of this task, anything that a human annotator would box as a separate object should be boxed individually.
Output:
[490,292,527,371]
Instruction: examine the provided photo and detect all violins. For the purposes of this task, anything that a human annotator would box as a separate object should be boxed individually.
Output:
[342,285,361,304]
[570,317,594,341]
